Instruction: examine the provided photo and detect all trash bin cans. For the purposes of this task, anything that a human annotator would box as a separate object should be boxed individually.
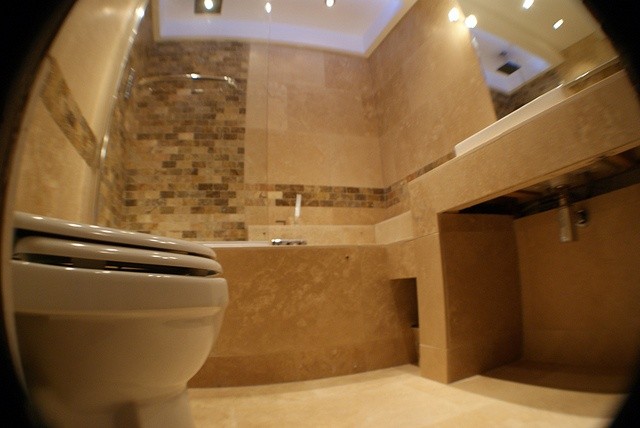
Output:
[410,324,419,365]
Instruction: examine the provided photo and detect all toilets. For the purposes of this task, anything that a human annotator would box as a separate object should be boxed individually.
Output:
[7,209,228,428]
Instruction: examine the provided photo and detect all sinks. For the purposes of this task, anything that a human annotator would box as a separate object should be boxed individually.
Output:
[455,84,563,154]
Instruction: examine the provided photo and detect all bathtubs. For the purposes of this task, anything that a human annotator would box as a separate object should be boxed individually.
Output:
[202,222,406,255]
